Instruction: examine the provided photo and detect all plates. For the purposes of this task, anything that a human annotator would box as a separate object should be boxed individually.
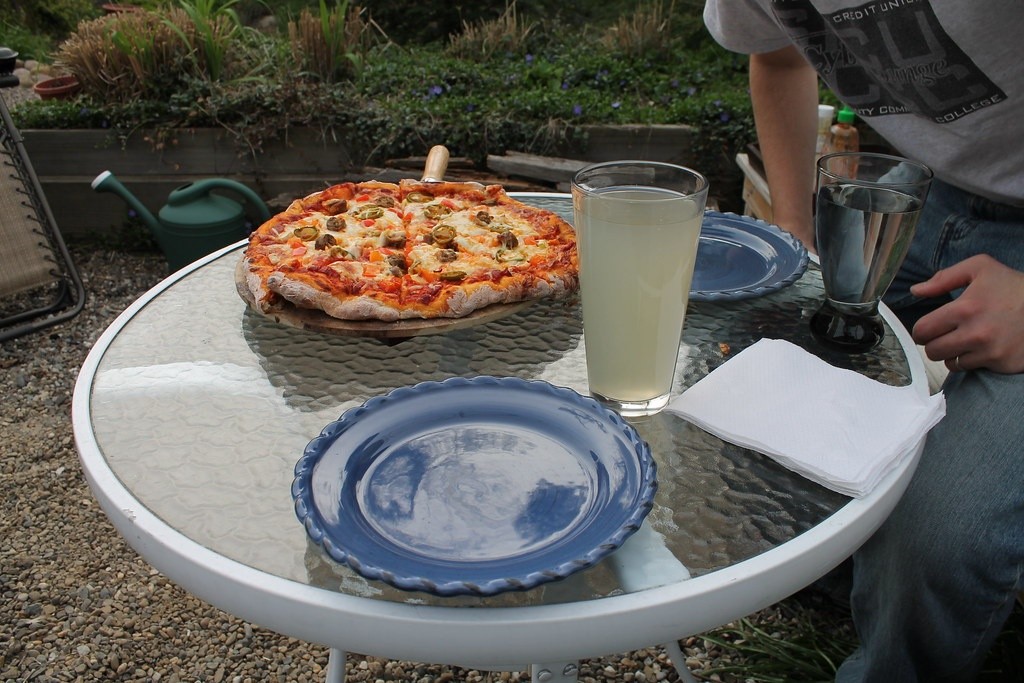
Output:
[682,209,812,306]
[290,375,658,602]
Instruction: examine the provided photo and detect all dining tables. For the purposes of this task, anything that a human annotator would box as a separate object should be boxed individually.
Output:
[73,193,929,683]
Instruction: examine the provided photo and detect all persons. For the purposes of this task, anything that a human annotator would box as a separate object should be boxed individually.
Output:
[702,0,1024,683]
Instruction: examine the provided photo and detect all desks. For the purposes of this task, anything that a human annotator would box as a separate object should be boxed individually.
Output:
[734,153,774,228]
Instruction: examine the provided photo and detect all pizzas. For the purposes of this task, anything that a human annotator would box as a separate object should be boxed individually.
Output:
[242,178,580,322]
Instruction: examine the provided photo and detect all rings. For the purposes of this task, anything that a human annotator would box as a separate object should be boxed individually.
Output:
[955,356,966,371]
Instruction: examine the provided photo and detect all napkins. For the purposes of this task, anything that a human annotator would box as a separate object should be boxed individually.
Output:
[664,337,947,499]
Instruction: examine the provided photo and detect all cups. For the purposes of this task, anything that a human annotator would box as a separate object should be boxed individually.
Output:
[571,160,708,416]
[815,147,933,346]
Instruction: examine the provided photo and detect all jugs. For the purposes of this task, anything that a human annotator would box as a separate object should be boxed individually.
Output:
[88,166,274,285]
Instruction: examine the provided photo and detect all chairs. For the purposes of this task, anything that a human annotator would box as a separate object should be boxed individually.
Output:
[0,93,86,339]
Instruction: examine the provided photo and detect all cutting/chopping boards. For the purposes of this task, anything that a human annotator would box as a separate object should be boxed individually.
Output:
[227,145,588,332]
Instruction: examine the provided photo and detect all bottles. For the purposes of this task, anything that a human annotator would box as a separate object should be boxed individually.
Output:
[817,107,861,198]
[812,104,834,192]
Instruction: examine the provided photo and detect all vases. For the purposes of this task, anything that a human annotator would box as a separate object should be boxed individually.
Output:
[34,76,81,100]
